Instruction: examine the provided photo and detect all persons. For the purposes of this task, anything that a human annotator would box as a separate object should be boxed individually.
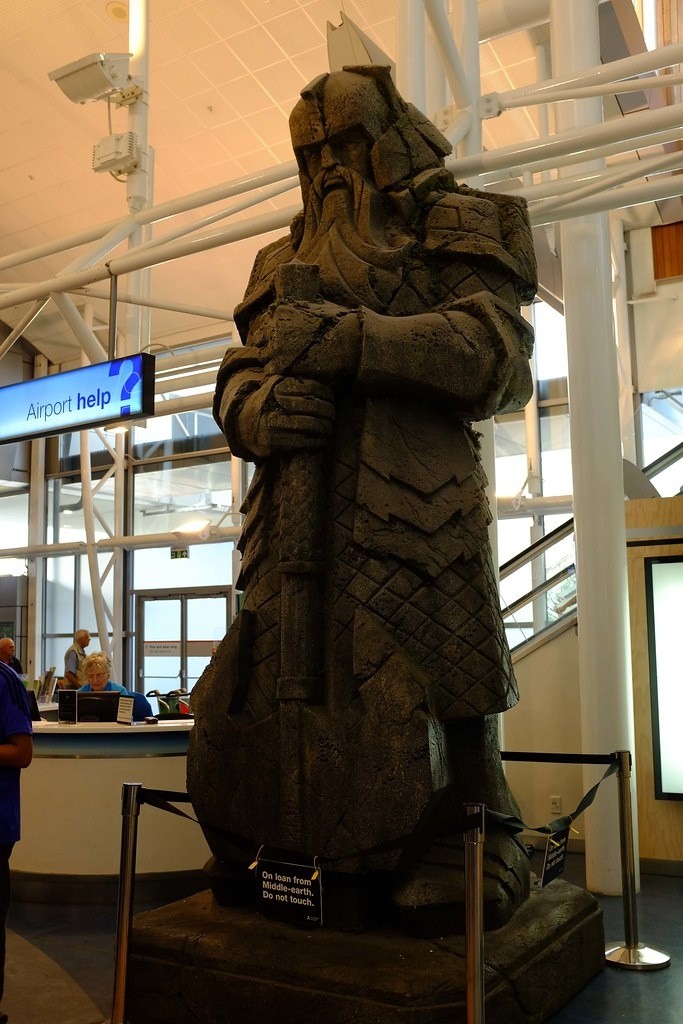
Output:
[75,651,129,695]
[64,630,91,690]
[184,64,531,930]
[0,661,35,1024]
[0,638,23,674]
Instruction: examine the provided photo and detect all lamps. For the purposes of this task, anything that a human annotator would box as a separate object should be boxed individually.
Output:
[46,51,144,108]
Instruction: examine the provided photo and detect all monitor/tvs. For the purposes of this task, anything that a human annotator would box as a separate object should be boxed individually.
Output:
[77,691,120,722]
[27,690,41,721]
